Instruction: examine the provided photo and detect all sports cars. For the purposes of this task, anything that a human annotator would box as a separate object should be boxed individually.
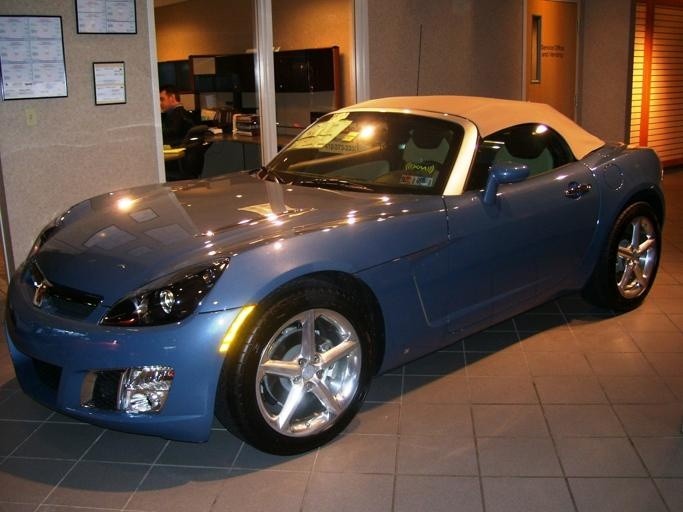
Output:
[5,93,664,457]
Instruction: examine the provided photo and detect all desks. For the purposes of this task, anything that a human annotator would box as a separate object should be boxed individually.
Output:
[209,118,310,146]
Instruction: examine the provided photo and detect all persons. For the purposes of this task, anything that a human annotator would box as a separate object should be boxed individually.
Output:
[159,84,188,144]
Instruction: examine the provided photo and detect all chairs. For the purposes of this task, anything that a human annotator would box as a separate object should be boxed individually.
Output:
[368,131,454,185]
[173,125,214,180]
[492,137,553,179]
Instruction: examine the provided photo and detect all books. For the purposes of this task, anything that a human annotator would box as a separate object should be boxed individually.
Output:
[201,108,260,137]
